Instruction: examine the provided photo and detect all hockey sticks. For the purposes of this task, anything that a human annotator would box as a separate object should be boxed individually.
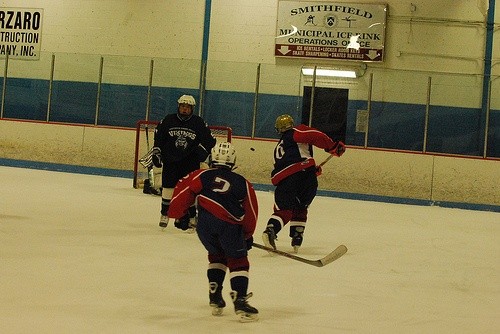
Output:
[145,127,161,194]
[318,145,343,168]
[187,223,347,267]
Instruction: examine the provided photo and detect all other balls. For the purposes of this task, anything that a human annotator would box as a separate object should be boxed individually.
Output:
[250,147,255,151]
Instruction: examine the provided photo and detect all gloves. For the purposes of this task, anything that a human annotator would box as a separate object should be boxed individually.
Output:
[325,139,347,157]
[175,214,191,231]
[316,165,322,176]
[151,150,162,168]
[192,145,208,162]
[246,235,254,251]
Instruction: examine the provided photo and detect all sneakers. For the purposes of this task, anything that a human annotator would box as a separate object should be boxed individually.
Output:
[262,224,277,256]
[206,282,226,317]
[228,291,260,323]
[291,226,306,255]
[158,214,170,232]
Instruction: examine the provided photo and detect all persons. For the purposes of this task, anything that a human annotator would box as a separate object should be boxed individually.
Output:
[262,115,346,256]
[168,142,260,323]
[152,94,217,234]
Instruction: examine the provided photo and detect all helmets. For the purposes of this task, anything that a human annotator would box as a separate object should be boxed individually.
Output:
[209,141,238,168]
[274,114,295,134]
[176,94,197,121]
